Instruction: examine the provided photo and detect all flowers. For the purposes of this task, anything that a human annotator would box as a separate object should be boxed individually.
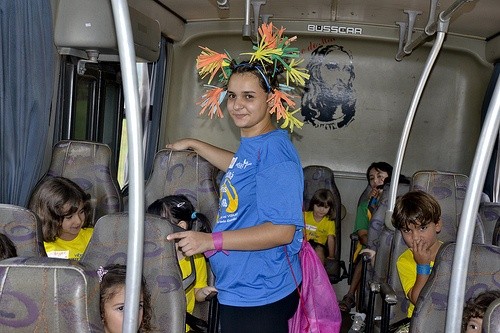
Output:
[196,22,310,134]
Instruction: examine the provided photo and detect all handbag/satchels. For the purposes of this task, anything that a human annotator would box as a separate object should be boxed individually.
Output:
[287,240,341,333]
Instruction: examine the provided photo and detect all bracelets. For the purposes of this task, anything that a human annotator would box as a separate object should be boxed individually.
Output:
[417,264,430,274]
[204,232,230,258]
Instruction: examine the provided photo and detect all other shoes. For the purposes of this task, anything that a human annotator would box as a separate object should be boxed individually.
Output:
[339,294,355,313]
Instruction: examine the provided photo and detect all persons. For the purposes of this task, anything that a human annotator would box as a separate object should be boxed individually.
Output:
[461,288,500,333]
[146,194,218,333]
[301,188,336,266]
[391,190,446,318]
[166,55,304,333]
[97,263,152,333]
[338,161,411,333]
[27,176,94,263]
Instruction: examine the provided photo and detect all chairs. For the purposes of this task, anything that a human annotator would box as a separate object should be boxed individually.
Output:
[348,171,500,333]
[302,166,341,284]
[0,139,221,333]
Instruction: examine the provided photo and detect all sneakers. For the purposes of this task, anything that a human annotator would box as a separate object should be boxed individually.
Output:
[348,312,366,333]
